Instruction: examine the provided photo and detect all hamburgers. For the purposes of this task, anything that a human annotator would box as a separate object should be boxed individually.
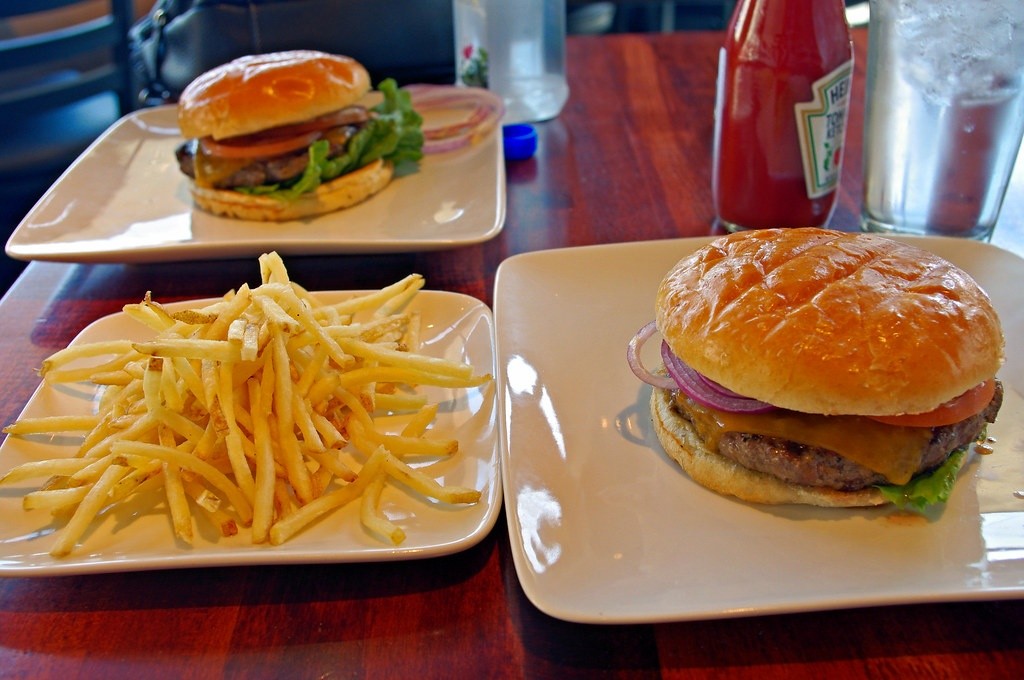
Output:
[177,48,425,217]
[625,226,1005,509]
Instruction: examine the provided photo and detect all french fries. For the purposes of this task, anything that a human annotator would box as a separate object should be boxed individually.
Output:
[1,250,494,563]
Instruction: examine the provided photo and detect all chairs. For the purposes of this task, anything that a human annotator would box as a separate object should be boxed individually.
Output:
[0,0,139,299]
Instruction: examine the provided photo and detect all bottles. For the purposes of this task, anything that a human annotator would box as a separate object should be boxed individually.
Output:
[452,0,570,125]
[712,0,855,236]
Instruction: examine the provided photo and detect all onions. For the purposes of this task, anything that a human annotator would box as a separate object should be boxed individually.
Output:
[397,80,506,153]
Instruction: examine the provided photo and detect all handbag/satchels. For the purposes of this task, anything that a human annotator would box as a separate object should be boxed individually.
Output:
[127,1,257,110]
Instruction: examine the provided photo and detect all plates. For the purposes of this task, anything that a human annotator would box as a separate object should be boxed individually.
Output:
[5,90,508,266]
[492,235,1024,623]
[0,289,503,580]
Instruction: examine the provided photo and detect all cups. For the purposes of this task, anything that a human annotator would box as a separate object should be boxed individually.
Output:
[858,0,1023,244]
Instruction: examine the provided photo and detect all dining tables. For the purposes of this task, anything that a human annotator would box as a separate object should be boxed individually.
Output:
[1,29,1024,679]
